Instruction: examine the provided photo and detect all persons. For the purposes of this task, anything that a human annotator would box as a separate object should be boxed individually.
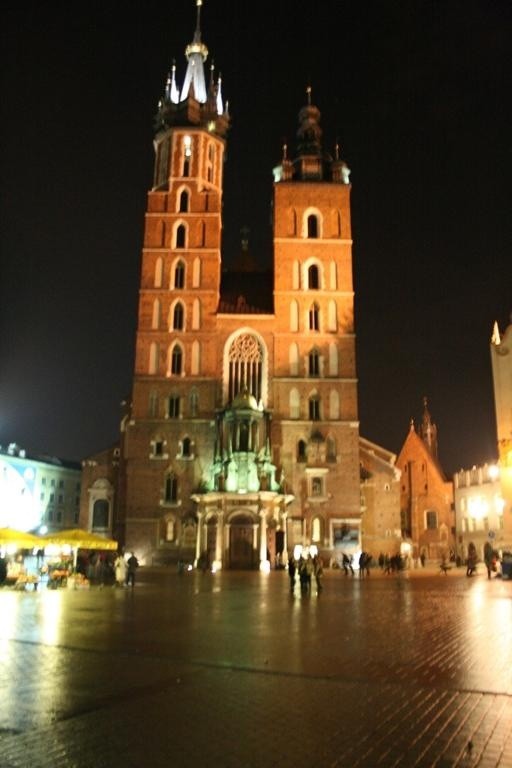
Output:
[124,551,139,586]
[288,541,512,592]
[113,550,130,587]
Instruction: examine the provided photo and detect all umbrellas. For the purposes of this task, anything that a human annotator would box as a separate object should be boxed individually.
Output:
[0,527,49,556]
[42,526,119,573]
